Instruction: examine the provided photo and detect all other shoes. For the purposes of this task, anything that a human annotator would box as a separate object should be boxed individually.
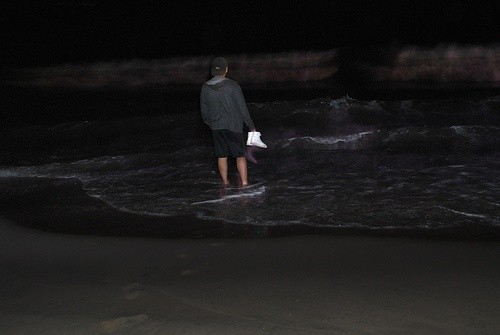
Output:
[246,132,267,148]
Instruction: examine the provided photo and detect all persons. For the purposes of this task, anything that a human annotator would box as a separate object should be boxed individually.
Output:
[200,57,257,188]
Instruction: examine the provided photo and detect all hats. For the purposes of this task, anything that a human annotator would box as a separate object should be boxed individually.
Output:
[211,57,228,75]
